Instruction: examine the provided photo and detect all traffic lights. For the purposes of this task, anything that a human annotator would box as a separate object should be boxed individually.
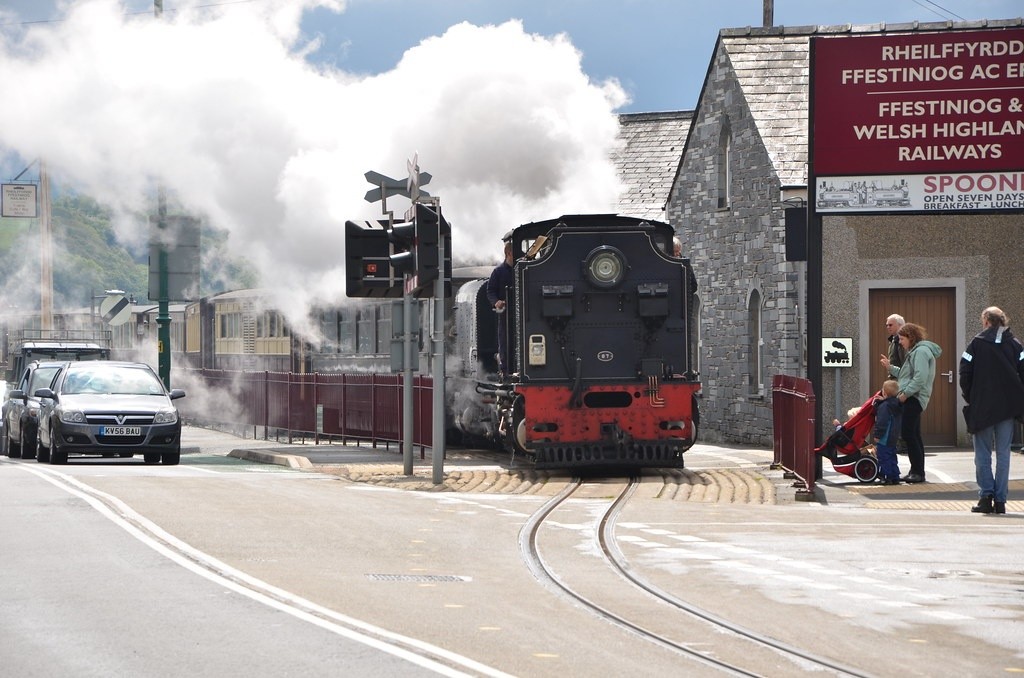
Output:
[386,202,439,295]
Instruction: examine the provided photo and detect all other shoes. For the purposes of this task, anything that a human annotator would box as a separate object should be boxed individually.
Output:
[993,502,1005,514]
[873,476,899,485]
[971,498,994,514]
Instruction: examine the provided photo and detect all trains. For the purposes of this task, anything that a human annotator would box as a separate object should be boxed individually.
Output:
[0,213,704,471]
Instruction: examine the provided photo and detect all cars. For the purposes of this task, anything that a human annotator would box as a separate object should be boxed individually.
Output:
[0,380,14,430]
[34,361,186,465]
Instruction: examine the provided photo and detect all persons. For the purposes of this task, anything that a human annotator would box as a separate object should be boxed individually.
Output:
[959,307,1024,513]
[673,236,698,292]
[487,242,514,374]
[873,380,901,485]
[880,323,942,483]
[813,407,862,453]
[886,314,908,381]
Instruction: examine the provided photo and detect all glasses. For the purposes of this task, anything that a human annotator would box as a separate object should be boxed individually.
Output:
[885,324,893,327]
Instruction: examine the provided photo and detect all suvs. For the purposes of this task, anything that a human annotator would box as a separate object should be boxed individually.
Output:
[5,328,112,381]
[5,360,68,459]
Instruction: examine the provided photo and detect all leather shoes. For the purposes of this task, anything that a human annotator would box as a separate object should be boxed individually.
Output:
[899,474,925,483]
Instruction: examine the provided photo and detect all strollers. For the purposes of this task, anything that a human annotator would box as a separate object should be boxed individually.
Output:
[814,387,906,483]
